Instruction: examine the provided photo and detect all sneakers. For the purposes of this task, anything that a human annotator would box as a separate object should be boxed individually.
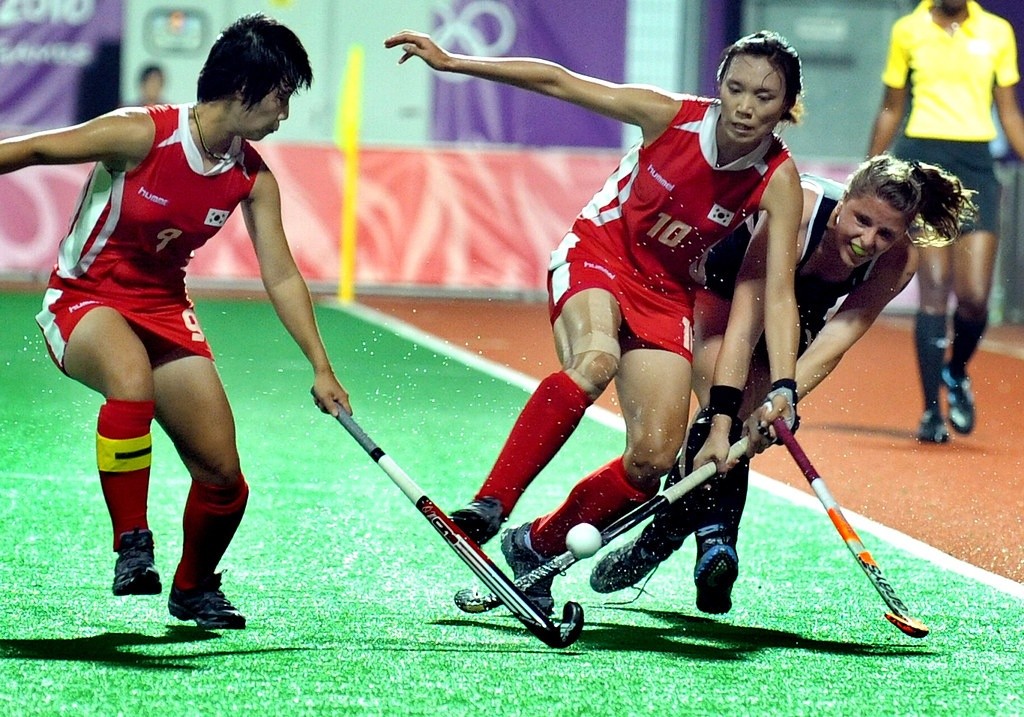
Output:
[168,569,247,629]
[449,497,502,546]
[918,409,947,442]
[112,527,162,595]
[501,522,566,616]
[694,527,739,614]
[590,520,684,605]
[942,364,974,434]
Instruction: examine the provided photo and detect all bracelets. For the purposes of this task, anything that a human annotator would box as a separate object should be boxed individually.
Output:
[710,385,743,422]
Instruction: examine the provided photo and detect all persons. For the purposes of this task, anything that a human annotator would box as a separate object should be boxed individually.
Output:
[0,13,353,630]
[384,30,980,619]
[865,0,1024,446]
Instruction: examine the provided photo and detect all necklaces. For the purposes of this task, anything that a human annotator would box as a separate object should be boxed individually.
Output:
[193,106,244,161]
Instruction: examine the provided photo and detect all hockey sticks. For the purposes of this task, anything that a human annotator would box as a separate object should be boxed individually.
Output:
[453,434,747,613]
[764,401,930,639]
[310,385,585,648]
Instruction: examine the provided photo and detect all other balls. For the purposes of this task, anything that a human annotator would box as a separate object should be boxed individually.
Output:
[565,522,602,559]
